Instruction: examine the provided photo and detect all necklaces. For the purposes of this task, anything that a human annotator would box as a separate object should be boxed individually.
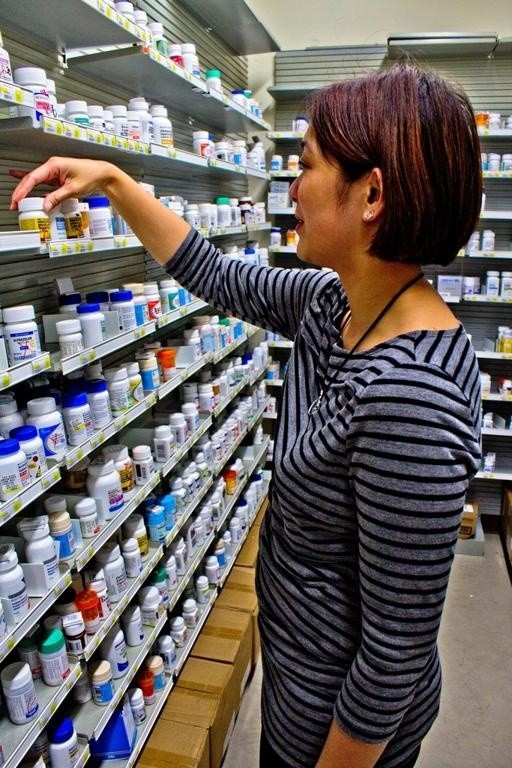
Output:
[307,270,426,417]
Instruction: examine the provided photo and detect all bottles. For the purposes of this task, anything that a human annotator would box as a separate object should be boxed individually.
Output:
[286,226,298,248]
[149,105,174,148]
[18,197,52,245]
[0,32,12,84]
[199,204,211,228]
[36,627,69,685]
[142,494,158,519]
[211,378,222,404]
[58,292,82,314]
[145,656,166,693]
[182,517,197,557]
[252,422,263,444]
[135,670,156,704]
[229,198,242,226]
[124,514,149,562]
[267,398,276,414]
[234,316,244,336]
[502,154,511,170]
[169,476,187,517]
[258,383,270,408]
[0,439,31,502]
[193,451,208,485]
[121,538,143,578]
[97,543,128,603]
[194,316,216,354]
[116,2,135,23]
[211,316,221,351]
[226,418,240,443]
[505,117,512,129]
[14,67,50,121]
[177,467,195,503]
[211,476,226,503]
[209,134,216,158]
[121,283,150,326]
[135,352,161,394]
[88,660,116,706]
[0,395,24,440]
[120,362,143,405]
[239,205,251,225]
[480,374,491,394]
[271,154,282,171]
[244,491,254,517]
[86,291,109,312]
[192,131,209,157]
[146,506,166,541]
[222,320,233,344]
[83,196,113,239]
[74,304,109,349]
[88,105,103,132]
[139,586,165,631]
[213,539,227,567]
[184,461,201,491]
[251,474,263,501]
[501,380,512,395]
[248,152,260,170]
[168,202,182,218]
[67,456,90,496]
[222,530,232,559]
[65,101,90,126]
[216,197,232,227]
[206,69,221,94]
[160,496,177,530]
[235,410,248,431]
[253,348,265,370]
[210,204,218,227]
[500,330,512,353]
[244,396,256,417]
[104,289,119,311]
[200,369,211,383]
[0,552,28,625]
[486,270,499,296]
[61,197,84,240]
[195,576,209,604]
[103,111,115,135]
[215,368,229,397]
[160,280,180,314]
[468,232,480,252]
[501,272,512,296]
[62,390,95,446]
[152,635,178,669]
[154,426,177,462]
[143,285,162,321]
[71,662,93,704]
[160,554,179,590]
[86,577,113,621]
[33,731,51,765]
[169,413,189,447]
[182,599,201,629]
[182,403,201,432]
[110,291,136,333]
[195,507,211,534]
[476,113,489,128]
[239,196,252,205]
[255,202,266,223]
[50,390,68,445]
[255,142,266,172]
[185,203,200,230]
[45,615,63,630]
[159,348,178,381]
[219,360,236,387]
[85,379,113,430]
[53,587,79,616]
[235,147,242,166]
[2,663,39,725]
[234,140,247,167]
[488,155,500,170]
[135,11,153,47]
[489,114,501,128]
[78,202,90,240]
[229,517,241,544]
[184,578,194,599]
[226,311,239,340]
[216,142,230,161]
[185,329,202,359]
[193,433,211,462]
[487,451,496,473]
[124,606,144,647]
[230,356,245,382]
[245,352,255,373]
[232,88,263,119]
[220,240,270,268]
[46,496,67,515]
[199,384,215,413]
[48,511,76,561]
[242,358,249,378]
[55,320,83,359]
[183,383,199,411]
[207,493,222,522]
[249,483,257,508]
[128,688,147,726]
[26,398,68,457]
[268,364,278,379]
[483,413,494,429]
[269,226,282,248]
[75,590,101,635]
[287,154,299,170]
[101,623,130,679]
[234,499,248,529]
[105,105,129,139]
[255,470,267,494]
[227,145,234,162]
[224,471,237,495]
[148,22,169,57]
[9,425,49,482]
[292,116,308,132]
[225,431,231,447]
[48,716,80,768]
[102,445,136,502]
[48,202,67,241]
[104,367,133,418]
[211,435,224,463]
[182,43,200,79]
[205,556,220,584]
[240,403,251,425]
[23,525,60,589]
[126,111,142,142]
[250,388,261,413]
[234,458,245,482]
[481,153,487,169]
[483,231,496,251]
[86,456,125,520]
[217,430,228,454]
[74,497,100,537]
[169,616,188,648]
[496,326,509,352]
[133,445,156,486]
[57,103,66,121]
[209,507,215,525]
[47,79,58,118]
[261,342,270,362]
[64,618,88,656]
[170,536,188,575]
[19,639,42,680]
[169,44,184,69]
[499,376,507,394]
[2,305,42,367]
[85,360,105,379]
[192,516,205,546]
[151,568,169,602]
[485,457,493,474]
[127,97,156,153]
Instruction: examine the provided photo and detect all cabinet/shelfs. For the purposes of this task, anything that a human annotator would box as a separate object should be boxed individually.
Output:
[269,42,512,534]
[1,0,273,768]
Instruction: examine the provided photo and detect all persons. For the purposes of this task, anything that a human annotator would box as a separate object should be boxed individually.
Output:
[9,64,482,768]
